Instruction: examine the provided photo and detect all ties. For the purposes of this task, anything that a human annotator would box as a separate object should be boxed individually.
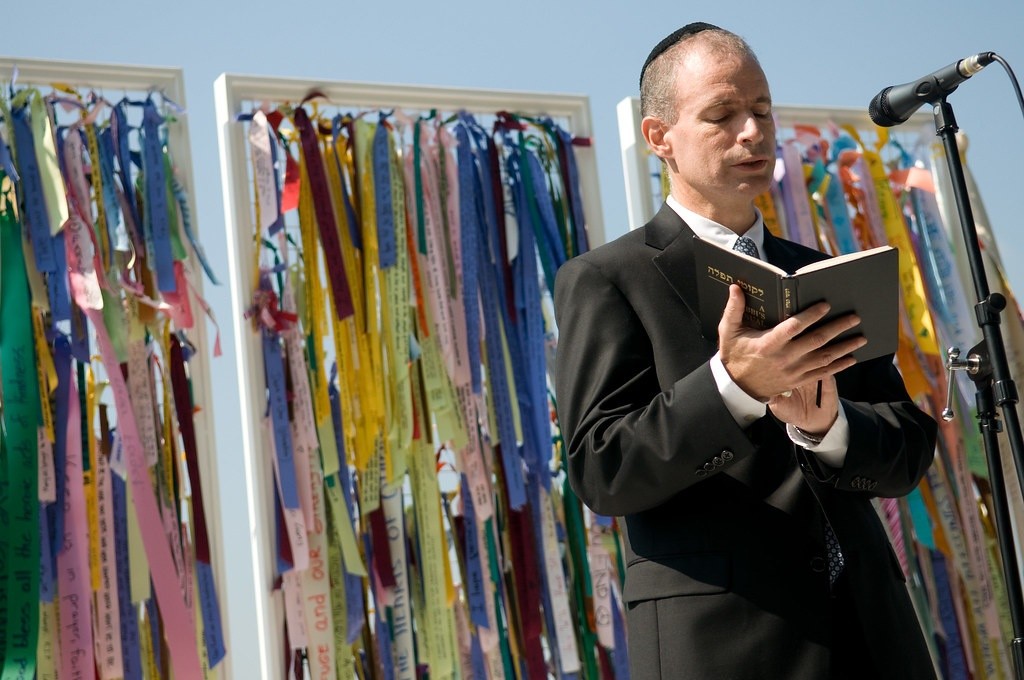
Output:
[737,234,844,587]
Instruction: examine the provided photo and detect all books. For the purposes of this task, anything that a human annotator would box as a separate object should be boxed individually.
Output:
[692,236,898,370]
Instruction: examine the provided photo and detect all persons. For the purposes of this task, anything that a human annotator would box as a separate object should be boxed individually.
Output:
[553,23,936,680]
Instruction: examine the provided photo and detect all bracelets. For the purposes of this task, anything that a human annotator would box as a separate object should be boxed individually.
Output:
[792,425,824,443]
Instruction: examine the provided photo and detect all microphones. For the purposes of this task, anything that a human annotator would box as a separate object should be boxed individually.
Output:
[868,51,997,127]
